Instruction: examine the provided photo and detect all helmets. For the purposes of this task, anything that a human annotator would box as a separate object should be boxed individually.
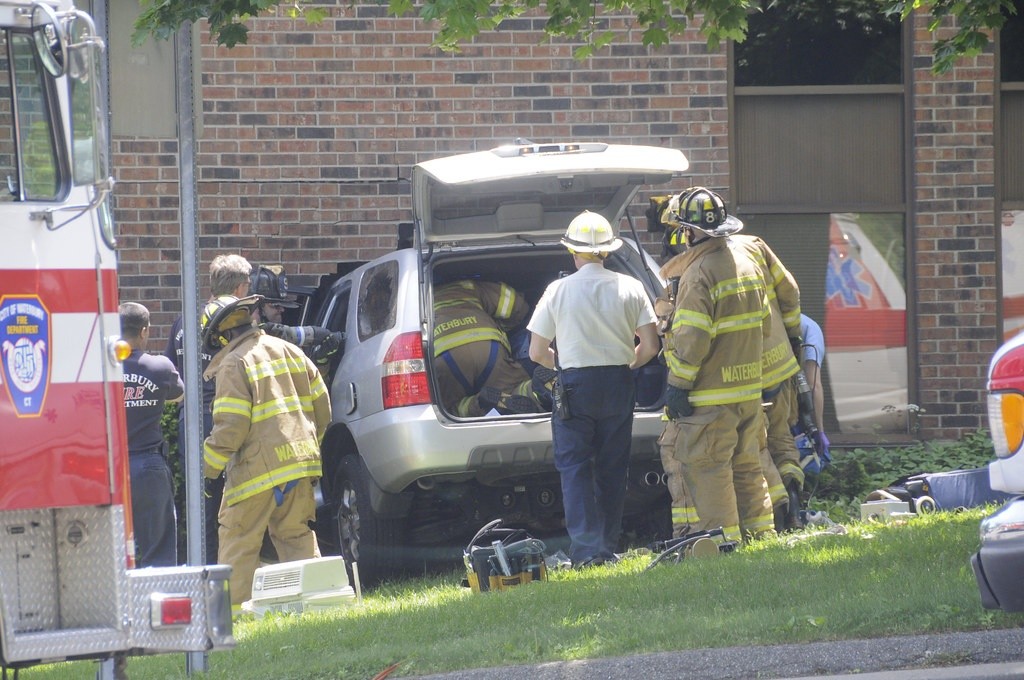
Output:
[461,518,553,590]
[558,209,624,260]
[197,291,265,354]
[660,185,744,239]
[250,261,302,310]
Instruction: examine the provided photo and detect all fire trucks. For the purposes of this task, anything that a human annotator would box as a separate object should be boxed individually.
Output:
[0,1,229,678]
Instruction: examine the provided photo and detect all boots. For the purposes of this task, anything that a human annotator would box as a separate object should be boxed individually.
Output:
[772,505,786,536]
[784,480,805,531]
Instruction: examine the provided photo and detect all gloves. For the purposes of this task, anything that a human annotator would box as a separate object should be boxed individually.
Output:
[204,477,217,499]
[316,333,341,360]
[662,383,697,421]
[819,431,830,453]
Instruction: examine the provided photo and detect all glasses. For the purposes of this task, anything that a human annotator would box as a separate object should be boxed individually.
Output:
[243,279,252,288]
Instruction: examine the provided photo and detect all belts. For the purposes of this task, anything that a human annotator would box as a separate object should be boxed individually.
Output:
[129,446,162,456]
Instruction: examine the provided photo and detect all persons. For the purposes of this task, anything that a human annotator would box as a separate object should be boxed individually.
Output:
[527,210,661,571]
[432,278,558,419]
[118,255,341,623]
[646,186,830,552]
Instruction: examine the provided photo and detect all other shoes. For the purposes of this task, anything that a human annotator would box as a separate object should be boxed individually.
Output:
[478,386,539,415]
[531,365,558,409]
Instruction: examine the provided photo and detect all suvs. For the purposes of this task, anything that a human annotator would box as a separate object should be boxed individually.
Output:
[268,143,709,576]
